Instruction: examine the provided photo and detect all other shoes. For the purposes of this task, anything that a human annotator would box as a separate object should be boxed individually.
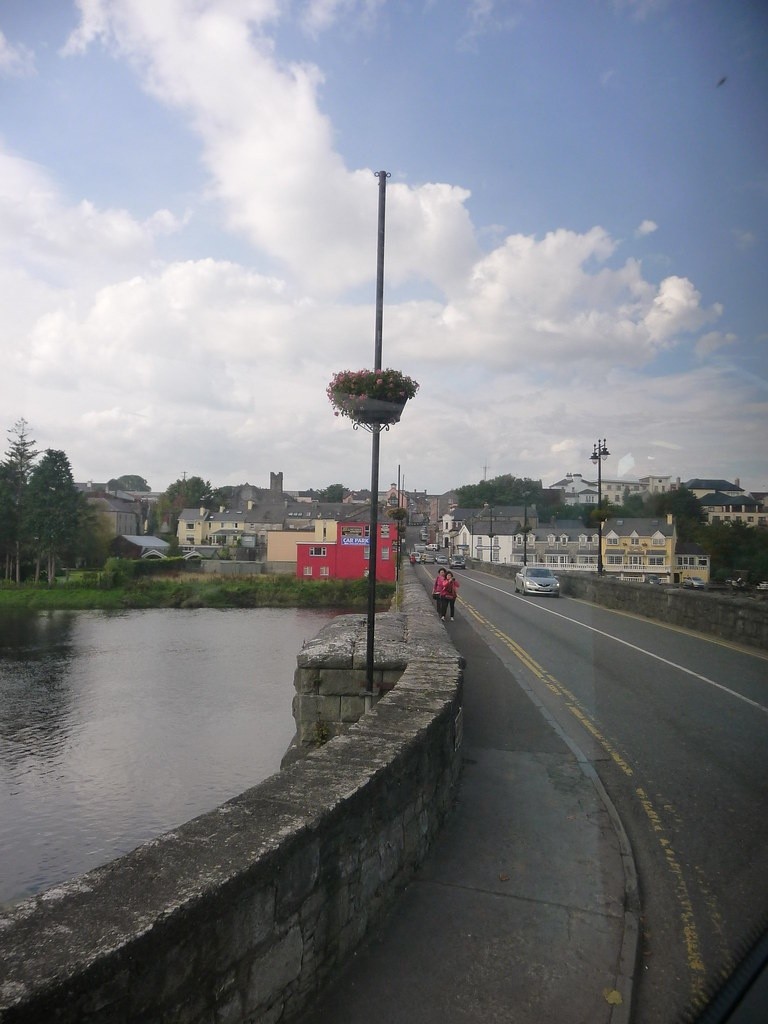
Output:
[441,616,445,622]
[451,617,454,621]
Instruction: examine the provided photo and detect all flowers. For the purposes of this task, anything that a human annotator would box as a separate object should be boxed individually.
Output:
[326,368,420,415]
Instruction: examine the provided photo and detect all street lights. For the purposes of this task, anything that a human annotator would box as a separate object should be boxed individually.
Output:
[588,438,612,578]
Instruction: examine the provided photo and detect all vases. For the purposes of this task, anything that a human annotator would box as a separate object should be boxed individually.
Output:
[363,398,403,422]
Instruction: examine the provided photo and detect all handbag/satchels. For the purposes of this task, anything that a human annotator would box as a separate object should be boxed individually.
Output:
[445,595,453,600]
[433,592,440,599]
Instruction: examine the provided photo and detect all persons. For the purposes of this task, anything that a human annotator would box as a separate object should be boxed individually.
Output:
[432,568,459,621]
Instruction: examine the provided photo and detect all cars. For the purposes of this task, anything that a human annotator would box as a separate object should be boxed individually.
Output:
[683,576,704,588]
[645,574,662,586]
[514,565,560,598]
[409,525,465,568]
